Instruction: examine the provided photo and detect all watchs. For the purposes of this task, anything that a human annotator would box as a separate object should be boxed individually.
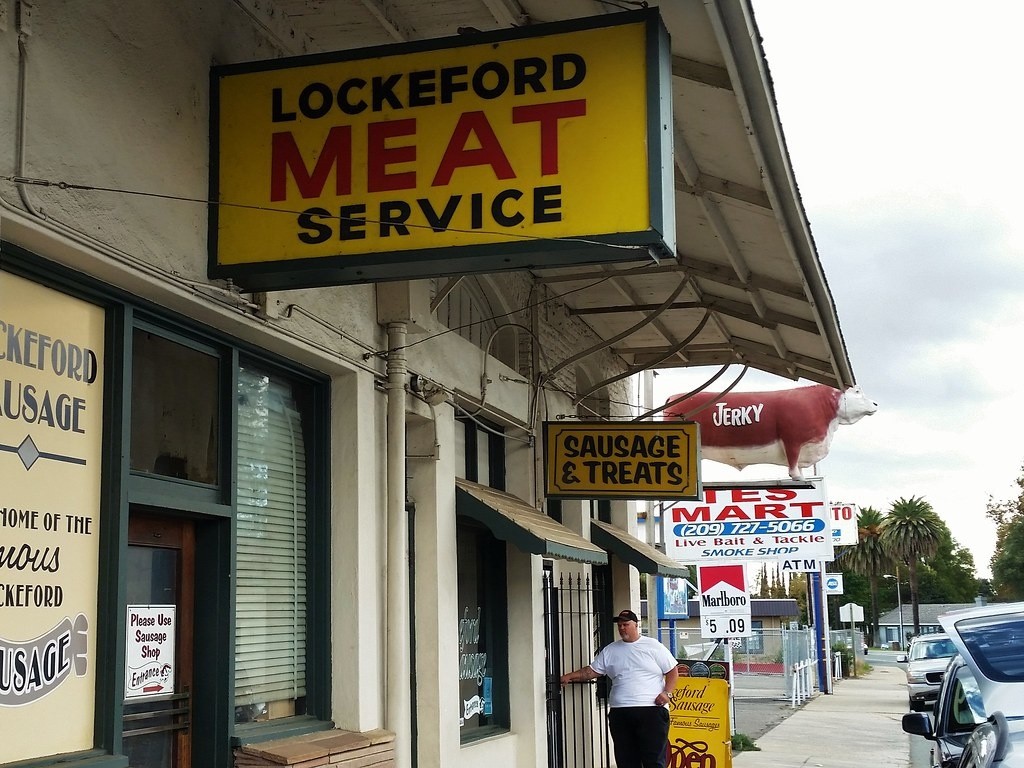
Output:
[664,691,673,698]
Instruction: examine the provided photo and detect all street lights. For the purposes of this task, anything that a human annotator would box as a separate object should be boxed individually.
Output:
[882,564,904,651]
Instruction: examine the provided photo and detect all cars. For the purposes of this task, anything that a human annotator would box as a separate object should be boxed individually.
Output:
[847,636,868,655]
[902,645,1024,768]
[936,601,1024,768]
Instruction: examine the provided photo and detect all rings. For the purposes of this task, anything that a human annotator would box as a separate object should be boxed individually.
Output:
[661,702,663,703]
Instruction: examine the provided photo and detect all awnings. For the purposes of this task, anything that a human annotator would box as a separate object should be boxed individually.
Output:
[455,477,609,566]
[641,599,799,617]
[590,518,690,578]
[240,1,860,392]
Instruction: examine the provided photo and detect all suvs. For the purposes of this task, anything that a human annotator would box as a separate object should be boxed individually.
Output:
[896,631,990,711]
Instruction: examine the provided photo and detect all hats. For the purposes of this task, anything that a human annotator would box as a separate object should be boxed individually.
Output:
[611,609,637,623]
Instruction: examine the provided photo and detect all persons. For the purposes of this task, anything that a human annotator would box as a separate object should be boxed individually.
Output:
[561,610,680,768]
